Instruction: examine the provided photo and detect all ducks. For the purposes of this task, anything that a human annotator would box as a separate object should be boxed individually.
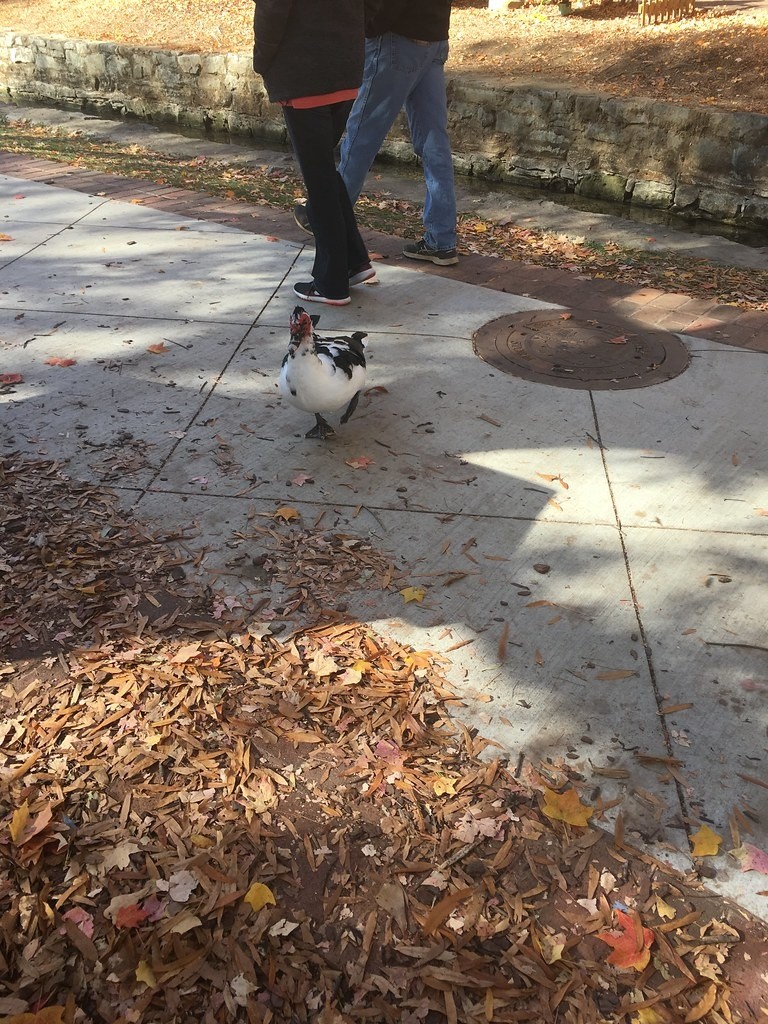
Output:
[277,304,370,439]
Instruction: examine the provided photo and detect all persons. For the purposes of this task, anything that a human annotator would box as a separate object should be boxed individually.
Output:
[294,0,460,265]
[254,0,376,305]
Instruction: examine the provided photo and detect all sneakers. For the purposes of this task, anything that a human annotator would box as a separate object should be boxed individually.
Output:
[293,283,351,305]
[404,238,460,265]
[293,205,314,236]
[347,263,376,285]
